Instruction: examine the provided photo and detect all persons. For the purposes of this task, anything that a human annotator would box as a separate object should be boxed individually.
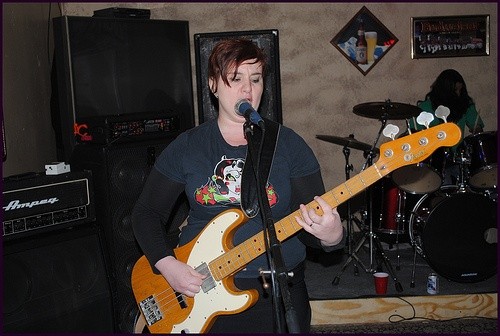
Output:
[131,40,344,336]
[408,69,483,152]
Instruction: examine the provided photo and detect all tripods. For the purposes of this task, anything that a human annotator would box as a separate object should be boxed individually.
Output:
[331,110,403,293]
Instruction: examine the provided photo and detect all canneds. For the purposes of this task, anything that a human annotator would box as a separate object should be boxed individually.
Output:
[427,273,440,295]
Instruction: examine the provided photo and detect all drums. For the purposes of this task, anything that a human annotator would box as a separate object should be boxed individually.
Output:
[408,186,500,270]
[393,129,452,194]
[364,168,411,243]
[453,130,500,188]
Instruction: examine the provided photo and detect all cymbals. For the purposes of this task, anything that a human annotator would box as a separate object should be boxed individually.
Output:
[352,101,423,120]
[315,135,380,153]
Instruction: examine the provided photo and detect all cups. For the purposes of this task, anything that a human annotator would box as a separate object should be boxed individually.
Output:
[365,32,377,62]
[373,272,389,294]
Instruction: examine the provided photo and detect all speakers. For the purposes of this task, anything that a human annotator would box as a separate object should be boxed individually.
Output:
[0,136,189,336]
[193,28,283,128]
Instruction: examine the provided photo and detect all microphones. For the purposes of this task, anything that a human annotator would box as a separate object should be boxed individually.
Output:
[234,99,265,131]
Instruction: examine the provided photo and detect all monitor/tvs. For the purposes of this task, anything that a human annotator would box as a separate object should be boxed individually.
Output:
[54,16,194,154]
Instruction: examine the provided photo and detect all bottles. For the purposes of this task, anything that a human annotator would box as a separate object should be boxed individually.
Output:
[357,22,366,64]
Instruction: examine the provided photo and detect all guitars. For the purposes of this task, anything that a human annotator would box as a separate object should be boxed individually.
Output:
[131,104,462,336]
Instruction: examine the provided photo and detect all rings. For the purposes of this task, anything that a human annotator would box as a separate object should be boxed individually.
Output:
[310,222,314,226]
